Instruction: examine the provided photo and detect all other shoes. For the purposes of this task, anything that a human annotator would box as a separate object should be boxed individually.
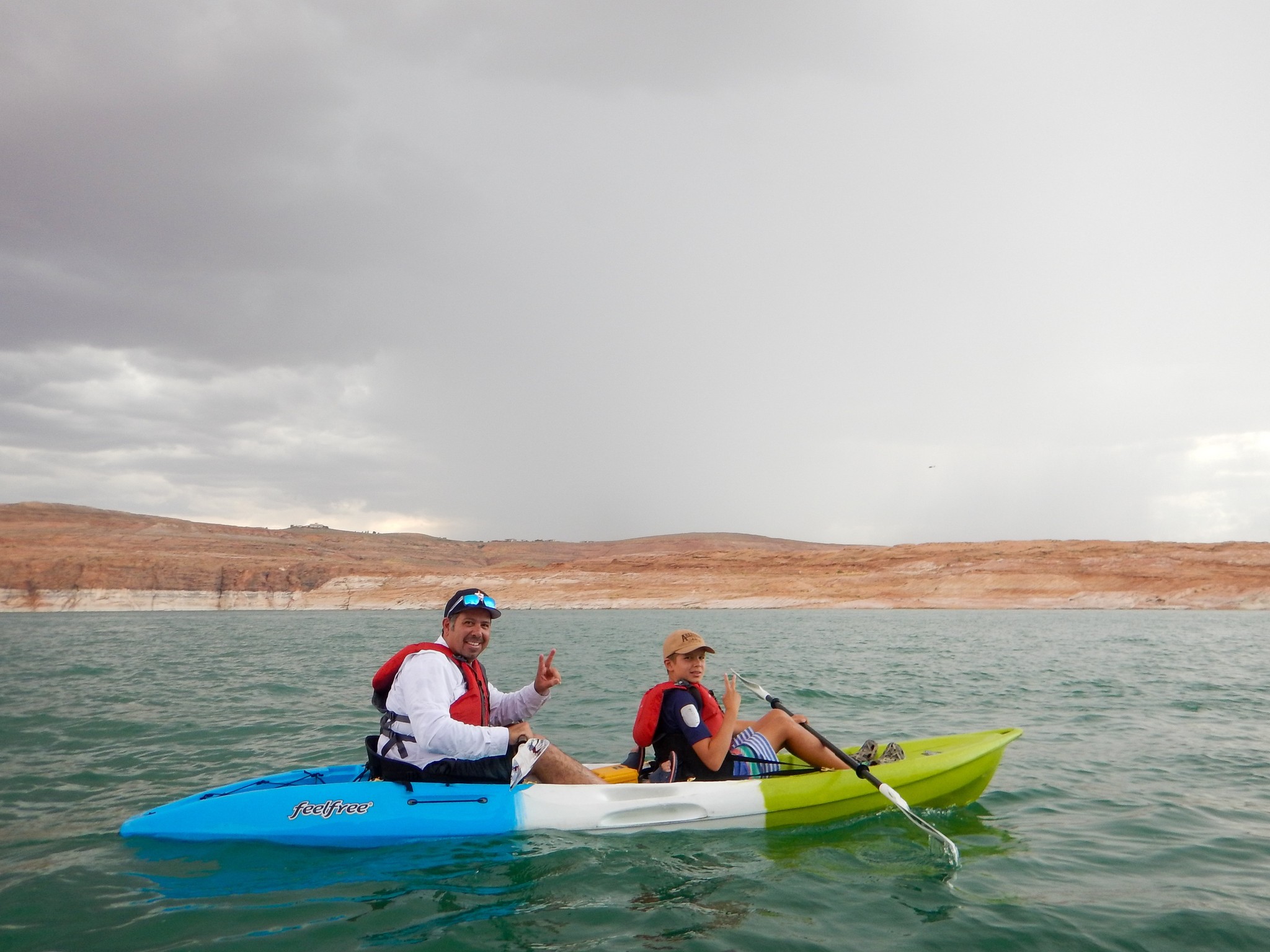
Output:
[872,742,905,766]
[851,739,878,763]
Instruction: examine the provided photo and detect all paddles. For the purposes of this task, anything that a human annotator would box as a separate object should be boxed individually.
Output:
[508,717,550,787]
[732,667,959,865]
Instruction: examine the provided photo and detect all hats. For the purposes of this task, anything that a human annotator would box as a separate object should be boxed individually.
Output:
[444,588,502,619]
[663,629,715,660]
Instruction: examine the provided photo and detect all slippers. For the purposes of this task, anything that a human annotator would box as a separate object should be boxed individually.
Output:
[647,750,678,783]
[621,743,645,779]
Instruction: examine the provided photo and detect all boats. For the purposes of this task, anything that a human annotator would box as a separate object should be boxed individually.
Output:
[118,722,1029,852]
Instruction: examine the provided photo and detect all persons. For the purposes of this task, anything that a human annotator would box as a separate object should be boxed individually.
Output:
[375,587,606,784]
[652,629,904,780]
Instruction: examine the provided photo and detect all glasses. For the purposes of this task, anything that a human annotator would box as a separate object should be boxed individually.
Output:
[447,594,496,619]
